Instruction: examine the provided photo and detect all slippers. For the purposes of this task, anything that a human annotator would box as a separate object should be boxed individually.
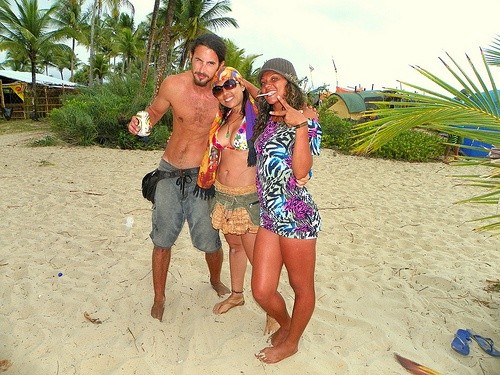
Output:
[451,328,472,355]
[472,334,500,357]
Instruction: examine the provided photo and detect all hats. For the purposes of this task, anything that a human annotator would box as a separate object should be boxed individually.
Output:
[257,58,302,89]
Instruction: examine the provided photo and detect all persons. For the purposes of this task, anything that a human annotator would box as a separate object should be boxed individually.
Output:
[131,34,319,322]
[212,66,284,336]
[242,57,321,365]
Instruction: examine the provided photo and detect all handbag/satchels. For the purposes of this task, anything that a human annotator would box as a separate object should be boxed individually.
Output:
[141,167,202,204]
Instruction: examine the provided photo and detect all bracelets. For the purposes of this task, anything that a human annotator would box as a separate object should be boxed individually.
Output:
[295,120,308,129]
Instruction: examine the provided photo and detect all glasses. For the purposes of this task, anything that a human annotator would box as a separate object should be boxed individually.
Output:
[210,78,241,98]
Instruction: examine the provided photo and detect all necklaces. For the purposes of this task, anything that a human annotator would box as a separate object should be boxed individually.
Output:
[226,115,242,134]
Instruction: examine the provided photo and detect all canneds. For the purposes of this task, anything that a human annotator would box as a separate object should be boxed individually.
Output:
[136,110,150,137]
